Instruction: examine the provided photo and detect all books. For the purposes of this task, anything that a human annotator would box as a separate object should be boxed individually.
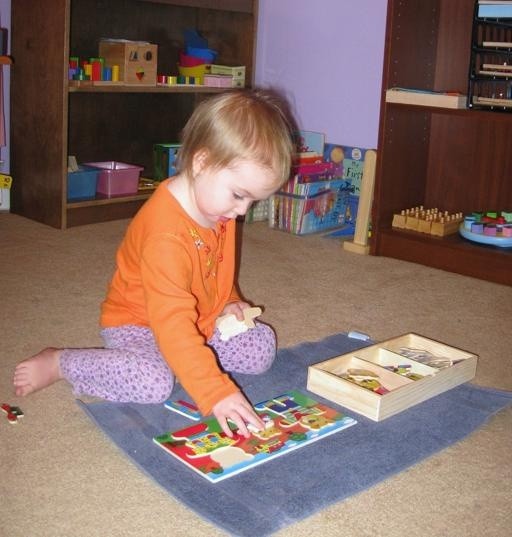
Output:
[270,146,352,233]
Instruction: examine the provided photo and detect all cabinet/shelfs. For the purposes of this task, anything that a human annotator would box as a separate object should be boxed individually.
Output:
[10,0,258,231]
[369,0,512,288]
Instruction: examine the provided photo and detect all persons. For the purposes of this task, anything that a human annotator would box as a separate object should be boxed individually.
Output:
[12,90,300,439]
[384,85,483,110]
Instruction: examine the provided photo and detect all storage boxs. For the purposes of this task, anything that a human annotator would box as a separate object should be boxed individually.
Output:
[84,160,145,196]
[96,33,158,91]
[66,165,101,199]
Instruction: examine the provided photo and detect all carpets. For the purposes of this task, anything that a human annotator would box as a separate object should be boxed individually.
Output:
[74,334,511,537]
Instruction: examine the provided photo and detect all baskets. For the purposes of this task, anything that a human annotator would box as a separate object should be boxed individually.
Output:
[271,188,354,234]
[247,198,270,224]
